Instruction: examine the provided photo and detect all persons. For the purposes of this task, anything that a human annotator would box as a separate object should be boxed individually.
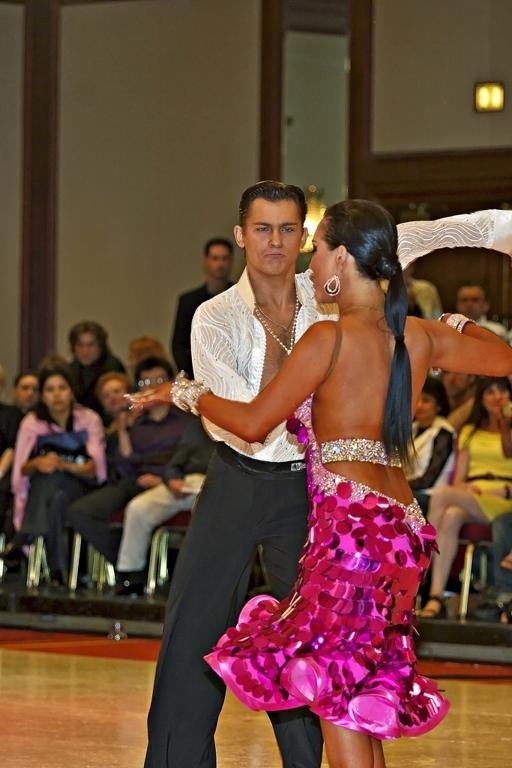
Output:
[141,180,510,767]
[404,279,512,621]
[168,238,247,380]
[1,321,212,598]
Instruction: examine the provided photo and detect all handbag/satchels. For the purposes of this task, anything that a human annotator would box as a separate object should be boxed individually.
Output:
[31,431,86,496]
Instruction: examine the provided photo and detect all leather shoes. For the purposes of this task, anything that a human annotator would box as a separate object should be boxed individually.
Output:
[420,594,445,619]
